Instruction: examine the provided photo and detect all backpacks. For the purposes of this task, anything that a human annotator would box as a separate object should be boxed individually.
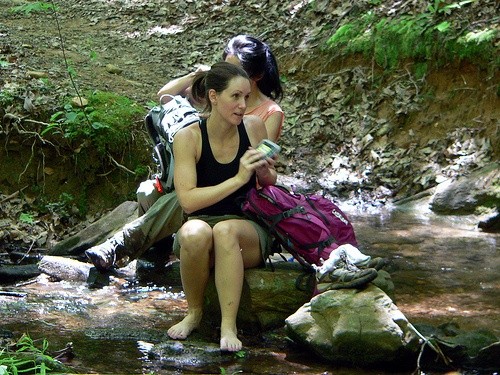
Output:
[241,183,357,294]
[144,94,201,193]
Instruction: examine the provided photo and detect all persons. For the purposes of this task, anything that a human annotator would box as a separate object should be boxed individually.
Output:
[85,36,284,272]
[167,62,279,351]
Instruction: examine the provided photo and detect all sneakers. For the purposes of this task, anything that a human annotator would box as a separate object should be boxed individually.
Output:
[320,244,383,272]
[85,236,122,273]
[312,248,377,291]
